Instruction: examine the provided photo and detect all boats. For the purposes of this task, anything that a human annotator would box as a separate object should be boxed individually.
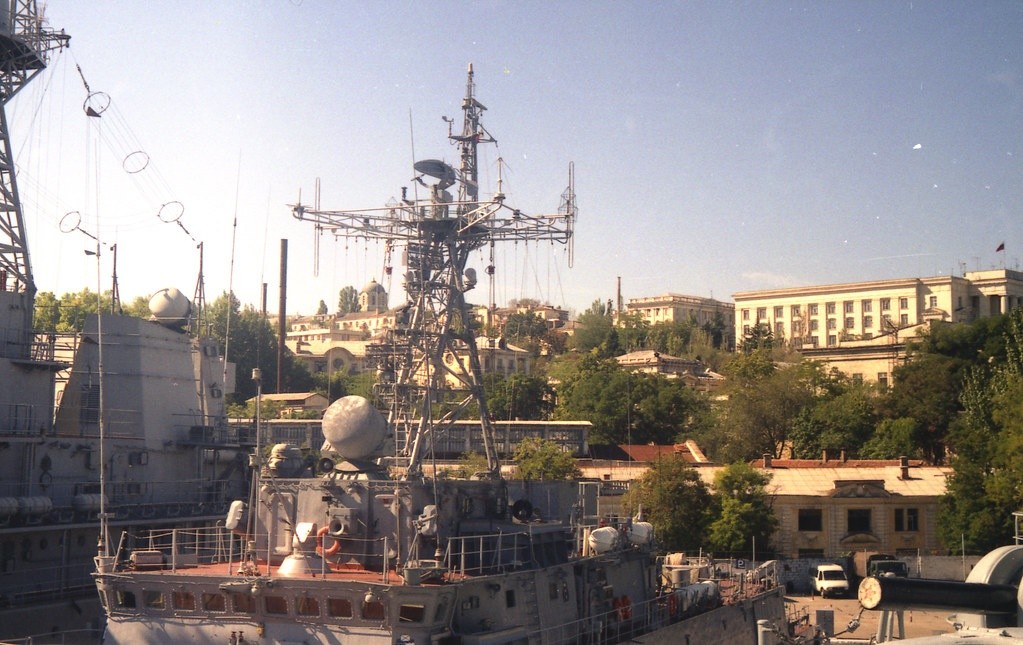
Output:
[84,61,830,645]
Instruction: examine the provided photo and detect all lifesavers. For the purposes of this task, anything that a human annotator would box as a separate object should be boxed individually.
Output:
[613,595,632,622]
[316,525,340,556]
[669,592,676,616]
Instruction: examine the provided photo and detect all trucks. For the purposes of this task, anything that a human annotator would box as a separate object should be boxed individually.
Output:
[849,551,910,578]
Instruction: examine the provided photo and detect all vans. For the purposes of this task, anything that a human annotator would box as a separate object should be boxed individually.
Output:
[809,563,849,599]
